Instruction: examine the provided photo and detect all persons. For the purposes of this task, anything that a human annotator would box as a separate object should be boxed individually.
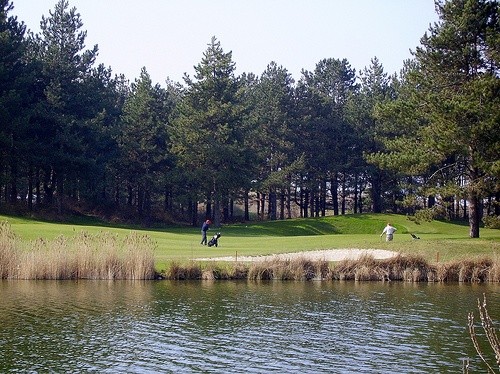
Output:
[379,223,397,242]
[199,218,212,245]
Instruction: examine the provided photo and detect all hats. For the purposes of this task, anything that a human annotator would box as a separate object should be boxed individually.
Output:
[206,220,211,224]
